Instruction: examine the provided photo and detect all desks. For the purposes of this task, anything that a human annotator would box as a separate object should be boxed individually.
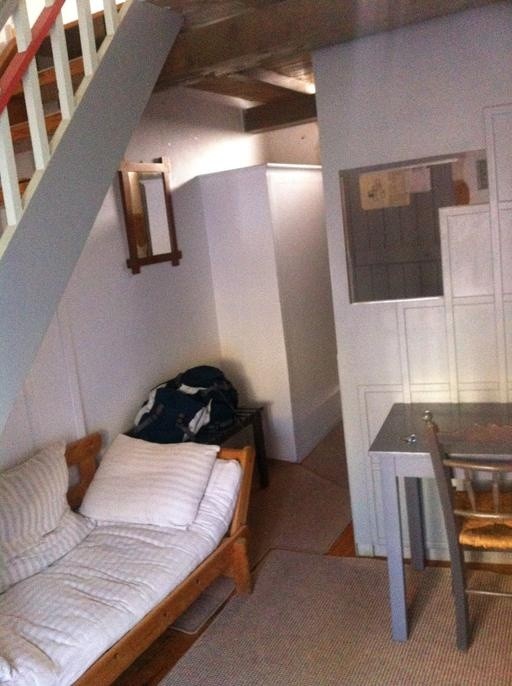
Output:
[369,400,512,643]
[197,403,270,489]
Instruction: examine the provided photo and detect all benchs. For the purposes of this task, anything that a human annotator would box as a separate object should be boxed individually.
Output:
[0,432,256,684]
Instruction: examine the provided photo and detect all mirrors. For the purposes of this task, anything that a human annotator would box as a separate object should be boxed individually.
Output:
[119,154,183,275]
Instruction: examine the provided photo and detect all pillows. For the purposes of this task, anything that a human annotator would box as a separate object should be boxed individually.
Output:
[77,433,221,533]
[0,438,96,594]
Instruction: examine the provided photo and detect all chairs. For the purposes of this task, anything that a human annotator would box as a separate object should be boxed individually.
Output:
[423,410,511,653]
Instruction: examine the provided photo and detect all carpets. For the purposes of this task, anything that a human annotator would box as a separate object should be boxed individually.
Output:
[305,421,351,492]
[251,456,357,558]
[171,544,270,637]
[158,546,512,685]
